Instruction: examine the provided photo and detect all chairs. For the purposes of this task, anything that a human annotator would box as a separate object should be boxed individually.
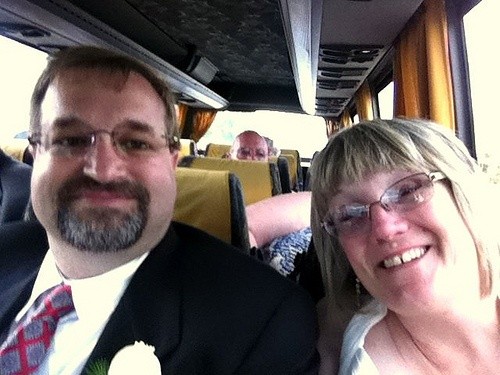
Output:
[203,143,303,193]
[176,155,280,205]
[167,167,250,251]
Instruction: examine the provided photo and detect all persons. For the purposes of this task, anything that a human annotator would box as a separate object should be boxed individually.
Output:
[0,46,321,375]
[226,130,268,161]
[309,115,500,375]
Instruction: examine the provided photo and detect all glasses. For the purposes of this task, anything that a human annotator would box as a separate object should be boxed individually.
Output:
[30,119,178,162]
[229,147,266,160]
[320,171,446,238]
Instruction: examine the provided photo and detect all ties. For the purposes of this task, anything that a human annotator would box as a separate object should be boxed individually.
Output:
[0,283,75,375]
